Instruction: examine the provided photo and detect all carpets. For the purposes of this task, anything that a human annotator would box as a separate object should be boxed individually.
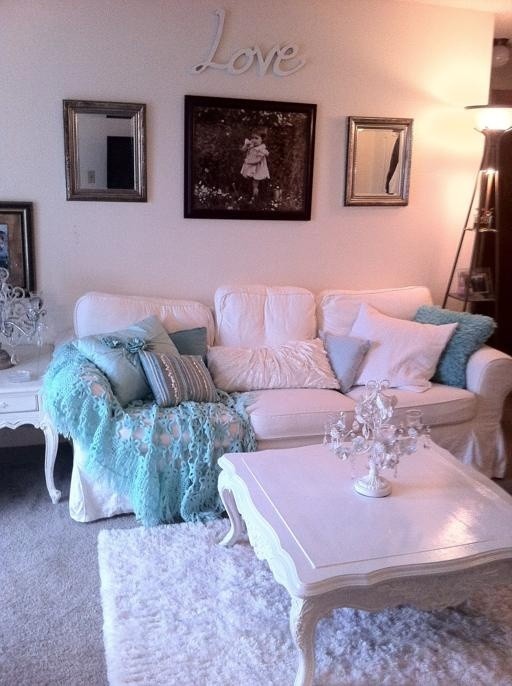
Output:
[94,517,512,681]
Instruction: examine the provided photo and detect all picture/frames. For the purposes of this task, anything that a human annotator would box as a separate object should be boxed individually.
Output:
[62,99,149,203]
[349,114,412,207]
[0,197,42,300]
[183,94,318,224]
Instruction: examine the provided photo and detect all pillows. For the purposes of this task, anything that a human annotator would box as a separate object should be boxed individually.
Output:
[170,321,207,371]
[139,347,223,407]
[72,315,190,401]
[322,329,374,397]
[414,302,497,387]
[350,301,457,395]
[208,337,342,393]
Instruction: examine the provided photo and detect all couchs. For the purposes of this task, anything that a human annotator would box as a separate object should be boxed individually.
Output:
[46,282,512,521]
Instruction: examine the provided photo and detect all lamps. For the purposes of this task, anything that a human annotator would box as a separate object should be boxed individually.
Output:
[439,101,512,328]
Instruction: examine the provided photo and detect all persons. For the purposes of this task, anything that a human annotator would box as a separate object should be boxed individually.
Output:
[238,124,271,203]
